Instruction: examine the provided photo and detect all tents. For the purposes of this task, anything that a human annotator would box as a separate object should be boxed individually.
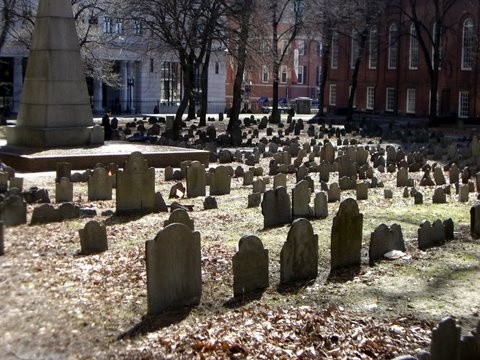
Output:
[312,98,319,105]
[278,97,287,106]
[258,96,270,106]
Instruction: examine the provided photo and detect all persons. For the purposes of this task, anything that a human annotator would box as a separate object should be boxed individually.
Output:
[153,105,159,114]
[103,109,114,139]
[115,102,131,116]
